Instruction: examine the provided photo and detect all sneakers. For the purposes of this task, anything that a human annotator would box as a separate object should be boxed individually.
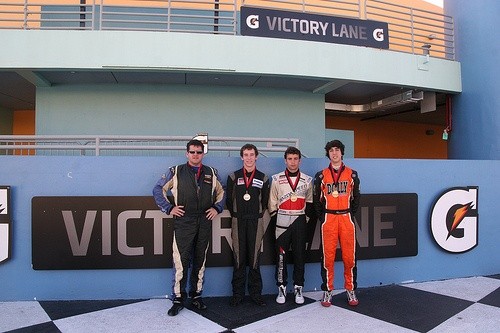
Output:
[293,285,304,304]
[322,291,332,307]
[191,297,206,310]
[346,290,359,306]
[276,285,287,304]
[168,298,184,316]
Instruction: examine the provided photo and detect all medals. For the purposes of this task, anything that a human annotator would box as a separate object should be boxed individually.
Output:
[331,190,339,198]
[243,193,251,201]
[290,194,297,202]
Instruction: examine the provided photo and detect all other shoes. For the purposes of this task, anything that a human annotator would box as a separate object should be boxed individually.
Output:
[249,292,266,306]
[231,293,247,307]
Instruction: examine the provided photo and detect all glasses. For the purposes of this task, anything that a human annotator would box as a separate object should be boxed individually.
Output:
[188,151,204,154]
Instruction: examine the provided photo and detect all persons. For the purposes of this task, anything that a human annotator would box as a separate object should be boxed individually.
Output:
[268,147,313,304]
[227,144,270,308]
[312,140,360,307]
[153,140,227,317]
